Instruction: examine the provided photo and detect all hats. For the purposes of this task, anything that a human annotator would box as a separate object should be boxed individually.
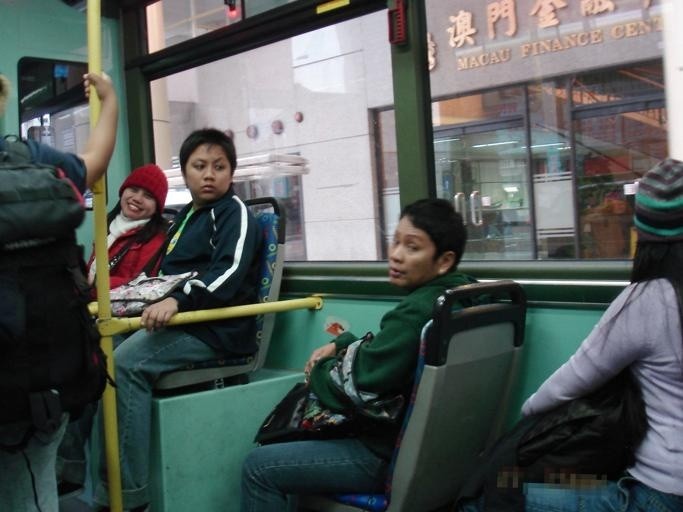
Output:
[119,163,168,214]
[633,158,683,237]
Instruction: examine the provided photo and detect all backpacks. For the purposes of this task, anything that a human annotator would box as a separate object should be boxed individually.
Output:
[2,140,107,434]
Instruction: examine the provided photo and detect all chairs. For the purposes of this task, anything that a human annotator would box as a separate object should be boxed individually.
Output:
[154,197,288,391]
[288,278,526,510]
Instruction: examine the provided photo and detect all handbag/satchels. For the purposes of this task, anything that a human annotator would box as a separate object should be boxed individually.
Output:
[485,371,646,478]
[255,383,367,444]
[110,272,200,317]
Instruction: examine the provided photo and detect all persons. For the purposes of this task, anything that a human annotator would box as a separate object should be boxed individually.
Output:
[49,126,264,511]
[238,196,484,510]
[0,65,120,511]
[453,157,683,511]
[80,161,170,302]
